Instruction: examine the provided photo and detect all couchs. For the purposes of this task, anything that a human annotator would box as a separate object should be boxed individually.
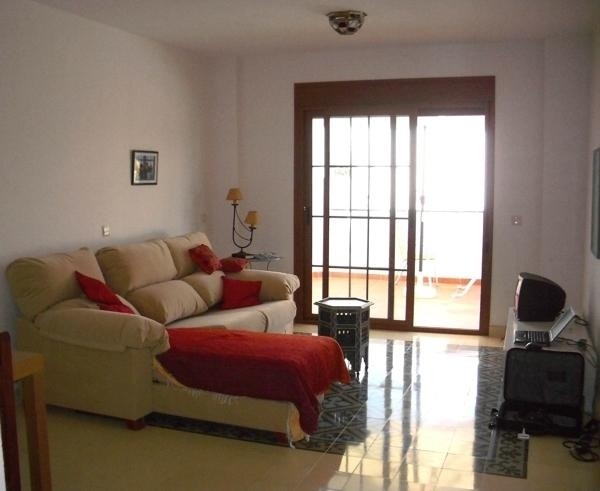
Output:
[16,269,351,447]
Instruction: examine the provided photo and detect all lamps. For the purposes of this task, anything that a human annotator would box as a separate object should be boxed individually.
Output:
[327,10,367,35]
[226,189,261,258]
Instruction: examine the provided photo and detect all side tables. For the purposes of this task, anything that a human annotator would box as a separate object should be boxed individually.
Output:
[233,256,285,270]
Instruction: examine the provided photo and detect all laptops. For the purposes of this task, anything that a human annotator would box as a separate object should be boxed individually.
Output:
[514,305,576,345]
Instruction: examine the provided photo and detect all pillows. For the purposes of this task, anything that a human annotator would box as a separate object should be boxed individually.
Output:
[6,232,262,322]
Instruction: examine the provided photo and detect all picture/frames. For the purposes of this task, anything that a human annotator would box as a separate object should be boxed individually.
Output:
[132,150,158,185]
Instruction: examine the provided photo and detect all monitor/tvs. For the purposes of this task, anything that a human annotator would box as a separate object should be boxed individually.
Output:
[514,270,565,319]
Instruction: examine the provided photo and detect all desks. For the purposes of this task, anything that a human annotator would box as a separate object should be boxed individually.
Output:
[497,307,585,439]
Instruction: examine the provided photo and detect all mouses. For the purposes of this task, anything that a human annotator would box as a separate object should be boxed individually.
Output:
[525,342,541,349]
